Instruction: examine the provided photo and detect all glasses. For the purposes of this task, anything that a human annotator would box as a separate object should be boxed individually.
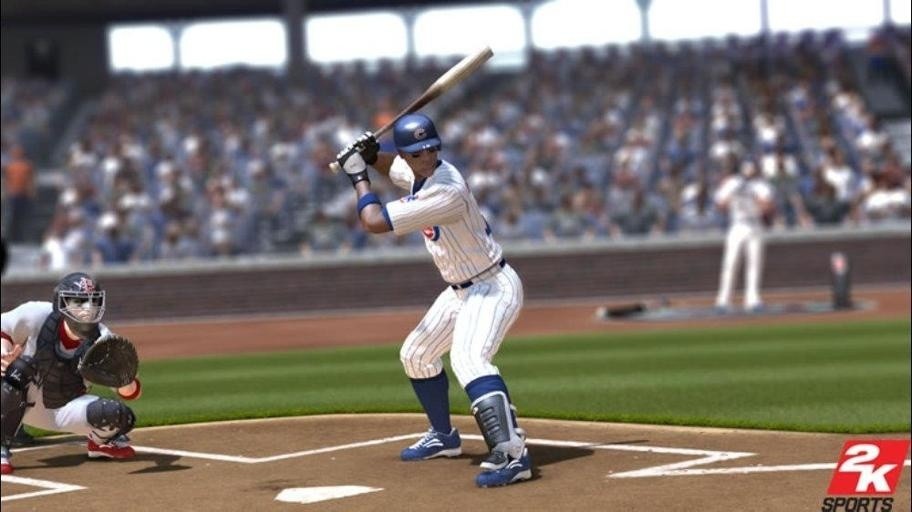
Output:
[410,147,443,156]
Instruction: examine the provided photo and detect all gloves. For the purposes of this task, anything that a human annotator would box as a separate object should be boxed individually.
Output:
[333,131,379,187]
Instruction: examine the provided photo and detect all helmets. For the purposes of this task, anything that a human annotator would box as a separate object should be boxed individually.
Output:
[52,274,107,328]
[392,113,445,156]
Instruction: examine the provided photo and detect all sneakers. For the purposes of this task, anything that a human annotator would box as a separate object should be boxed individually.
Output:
[401,413,462,464]
[0,443,11,474]
[84,436,137,459]
[468,456,535,489]
